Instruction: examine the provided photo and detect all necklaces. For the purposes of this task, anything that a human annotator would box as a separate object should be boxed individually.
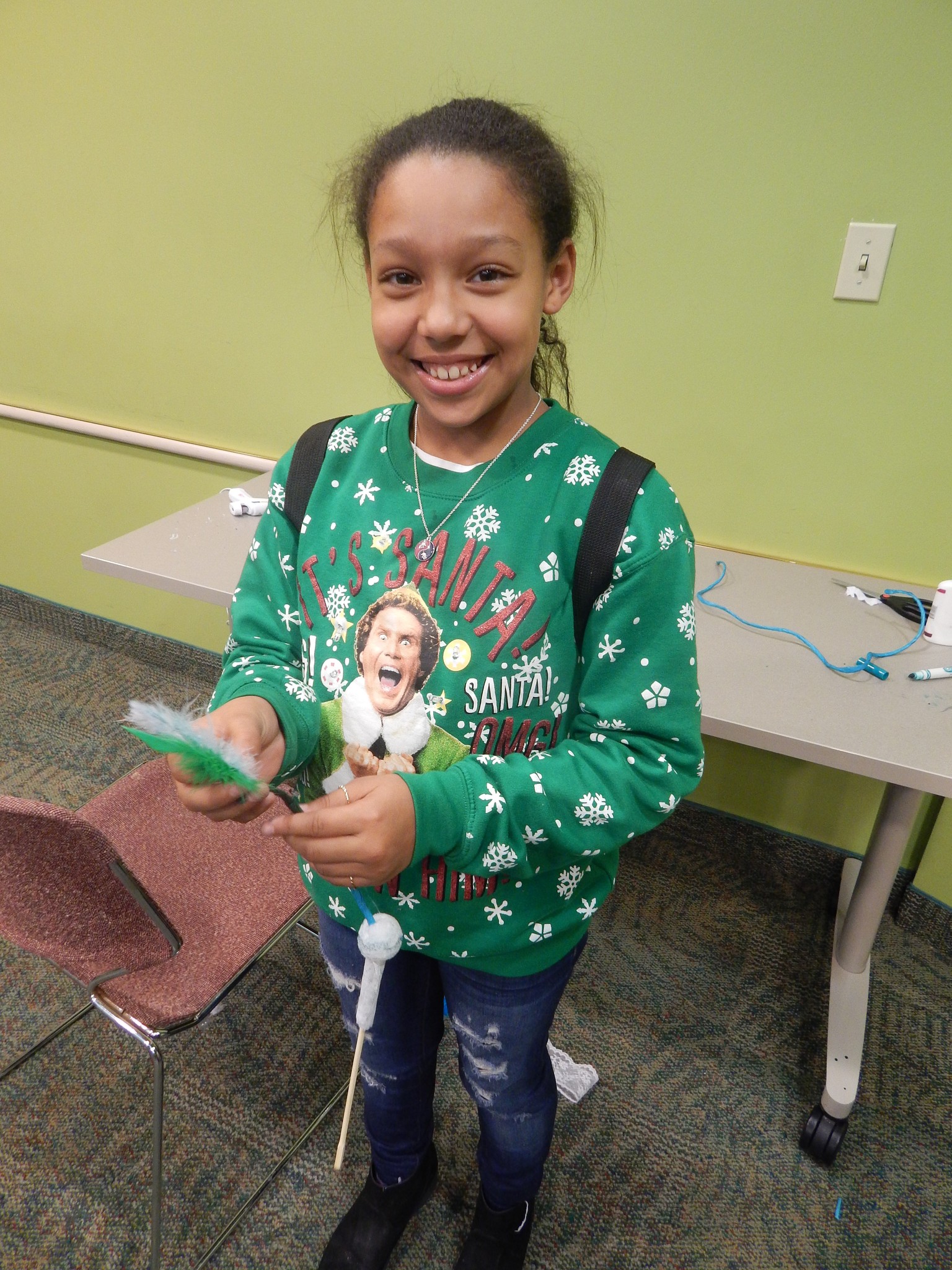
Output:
[413,392,541,561]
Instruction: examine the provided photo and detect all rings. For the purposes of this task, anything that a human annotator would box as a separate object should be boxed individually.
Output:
[338,784,350,804]
[349,876,356,890]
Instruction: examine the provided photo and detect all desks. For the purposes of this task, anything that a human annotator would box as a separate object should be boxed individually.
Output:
[82,471,952,1163]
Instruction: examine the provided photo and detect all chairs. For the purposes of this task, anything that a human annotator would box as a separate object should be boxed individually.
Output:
[0,755,352,1270]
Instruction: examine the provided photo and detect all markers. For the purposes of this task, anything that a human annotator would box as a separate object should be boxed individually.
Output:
[909,667,952,680]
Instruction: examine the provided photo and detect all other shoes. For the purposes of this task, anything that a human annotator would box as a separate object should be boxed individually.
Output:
[450,1180,534,1270]
[317,1139,437,1270]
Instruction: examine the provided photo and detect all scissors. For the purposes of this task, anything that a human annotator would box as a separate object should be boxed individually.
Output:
[829,577,933,625]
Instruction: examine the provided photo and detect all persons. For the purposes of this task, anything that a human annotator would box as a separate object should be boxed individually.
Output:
[164,93,703,1270]
